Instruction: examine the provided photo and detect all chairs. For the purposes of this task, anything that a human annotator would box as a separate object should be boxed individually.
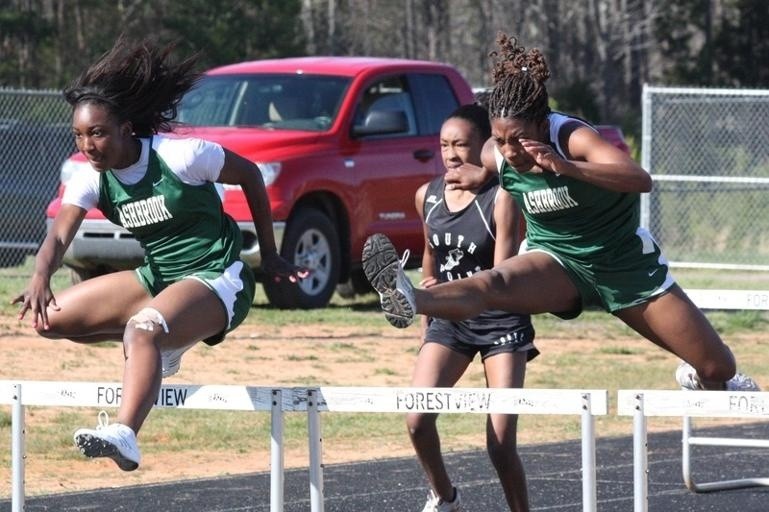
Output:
[268,99,306,121]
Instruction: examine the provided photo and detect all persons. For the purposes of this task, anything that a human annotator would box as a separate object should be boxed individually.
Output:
[358,25,764,394]
[10,31,316,473]
[404,102,541,512]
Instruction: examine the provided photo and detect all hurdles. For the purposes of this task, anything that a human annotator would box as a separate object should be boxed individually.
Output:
[619,388,769,511]
[0,381,607,511]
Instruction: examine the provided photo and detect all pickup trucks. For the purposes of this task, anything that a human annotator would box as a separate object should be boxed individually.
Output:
[46,54,632,308]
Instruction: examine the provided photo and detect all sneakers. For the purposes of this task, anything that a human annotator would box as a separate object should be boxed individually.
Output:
[161,345,180,378]
[362,233,417,329]
[73,423,139,471]
[422,487,461,512]
[676,363,758,391]
